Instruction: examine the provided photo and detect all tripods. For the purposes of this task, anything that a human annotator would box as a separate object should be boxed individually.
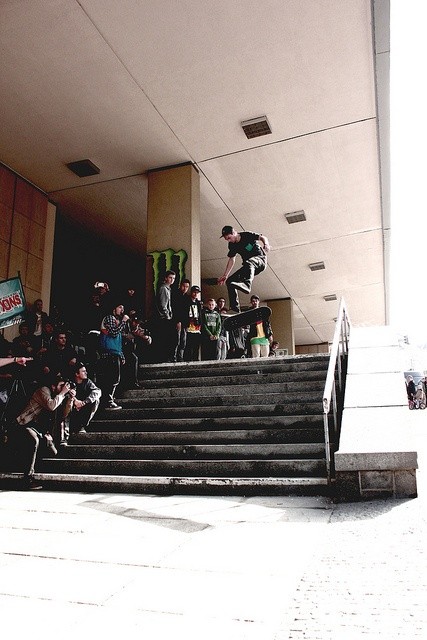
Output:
[0,379,28,430]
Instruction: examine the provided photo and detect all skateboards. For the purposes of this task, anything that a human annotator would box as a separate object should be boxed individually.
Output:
[223,306,272,331]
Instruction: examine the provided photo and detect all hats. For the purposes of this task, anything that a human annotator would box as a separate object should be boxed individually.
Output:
[220,225,233,238]
[191,285,202,292]
[73,362,90,372]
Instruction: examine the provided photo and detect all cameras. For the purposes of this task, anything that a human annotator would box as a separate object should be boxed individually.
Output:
[66,379,76,389]
[143,328,152,335]
[127,314,147,327]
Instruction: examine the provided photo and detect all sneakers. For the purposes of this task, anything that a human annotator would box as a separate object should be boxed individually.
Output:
[222,310,242,318]
[103,402,122,411]
[231,281,250,293]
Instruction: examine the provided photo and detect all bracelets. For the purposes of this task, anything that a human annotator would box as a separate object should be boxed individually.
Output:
[14,358,18,364]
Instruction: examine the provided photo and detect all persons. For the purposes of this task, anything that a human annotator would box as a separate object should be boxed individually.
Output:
[94,280,110,324]
[216,297,229,359]
[219,307,227,317]
[187,284,200,361]
[405,374,427,409]
[29,298,48,343]
[17,379,65,491]
[123,314,154,389]
[215,224,272,319]
[59,363,102,446]
[202,298,222,360]
[45,322,58,342]
[229,322,246,359]
[173,278,190,362]
[155,270,174,362]
[99,304,130,411]
[122,284,139,313]
[0,353,20,381]
[43,333,75,381]
[0,356,34,367]
[247,294,273,359]
[271,341,282,358]
[14,324,32,349]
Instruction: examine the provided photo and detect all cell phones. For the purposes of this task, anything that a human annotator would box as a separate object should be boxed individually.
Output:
[98,283,104,287]
